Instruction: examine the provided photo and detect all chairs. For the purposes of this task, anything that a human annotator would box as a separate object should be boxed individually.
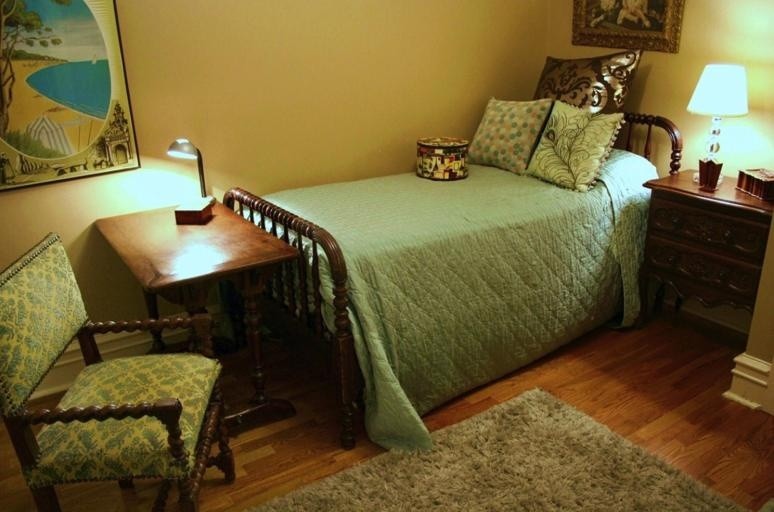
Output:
[0,234,234,512]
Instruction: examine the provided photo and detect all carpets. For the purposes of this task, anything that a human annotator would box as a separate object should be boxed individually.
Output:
[233,390,747,512]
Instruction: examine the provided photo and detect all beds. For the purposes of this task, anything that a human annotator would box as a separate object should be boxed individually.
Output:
[224,108,682,446]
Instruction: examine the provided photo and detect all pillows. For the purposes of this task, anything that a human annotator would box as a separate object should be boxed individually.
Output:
[531,49,641,111]
[526,100,626,192]
[468,96,550,174]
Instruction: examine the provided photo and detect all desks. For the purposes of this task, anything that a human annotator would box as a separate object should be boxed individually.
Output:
[95,199,304,435]
[638,168,774,325]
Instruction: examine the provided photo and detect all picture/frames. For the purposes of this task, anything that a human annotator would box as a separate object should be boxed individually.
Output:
[0,0,141,193]
[570,0,685,54]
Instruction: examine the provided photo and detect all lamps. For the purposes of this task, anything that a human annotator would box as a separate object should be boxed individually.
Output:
[169,135,215,225]
[687,63,751,188]
